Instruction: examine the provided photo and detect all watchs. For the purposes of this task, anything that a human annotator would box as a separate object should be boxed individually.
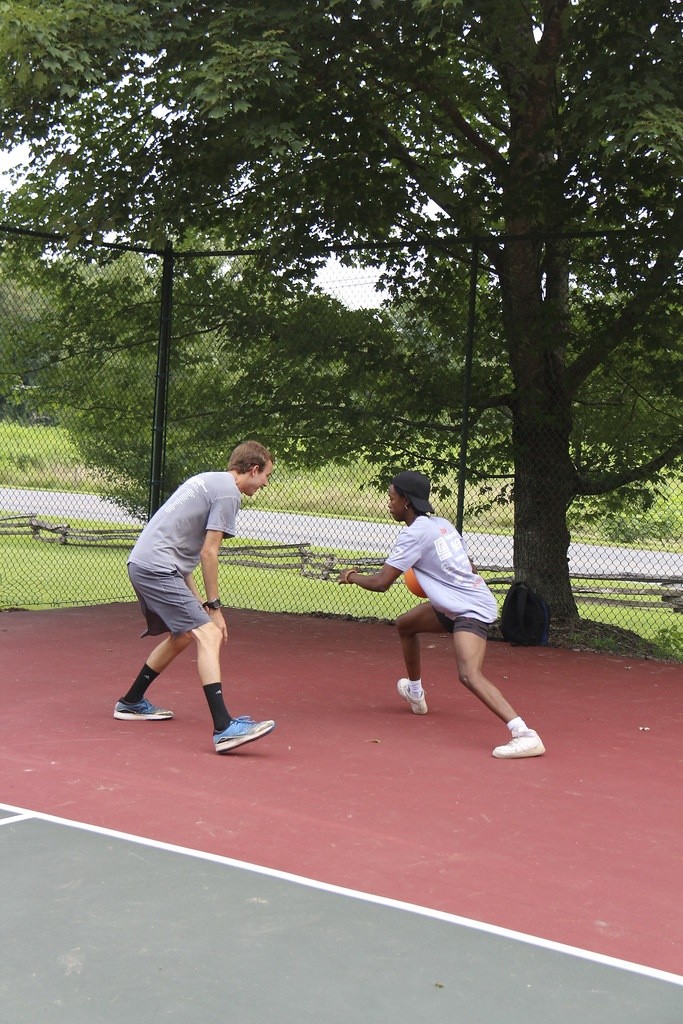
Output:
[202,598,222,610]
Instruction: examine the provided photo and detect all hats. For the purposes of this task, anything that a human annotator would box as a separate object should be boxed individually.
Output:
[391,471,436,514]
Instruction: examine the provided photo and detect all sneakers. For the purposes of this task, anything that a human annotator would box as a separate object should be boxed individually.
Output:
[492,728,546,759]
[112,697,174,721]
[396,677,429,715]
[213,715,276,754]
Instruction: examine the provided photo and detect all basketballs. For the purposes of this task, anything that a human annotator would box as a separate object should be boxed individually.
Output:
[404,566,428,598]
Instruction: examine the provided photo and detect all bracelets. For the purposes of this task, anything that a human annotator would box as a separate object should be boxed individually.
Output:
[345,570,357,585]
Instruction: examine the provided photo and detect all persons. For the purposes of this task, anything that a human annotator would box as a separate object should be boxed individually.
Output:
[113,441,276,755]
[337,471,546,760]
